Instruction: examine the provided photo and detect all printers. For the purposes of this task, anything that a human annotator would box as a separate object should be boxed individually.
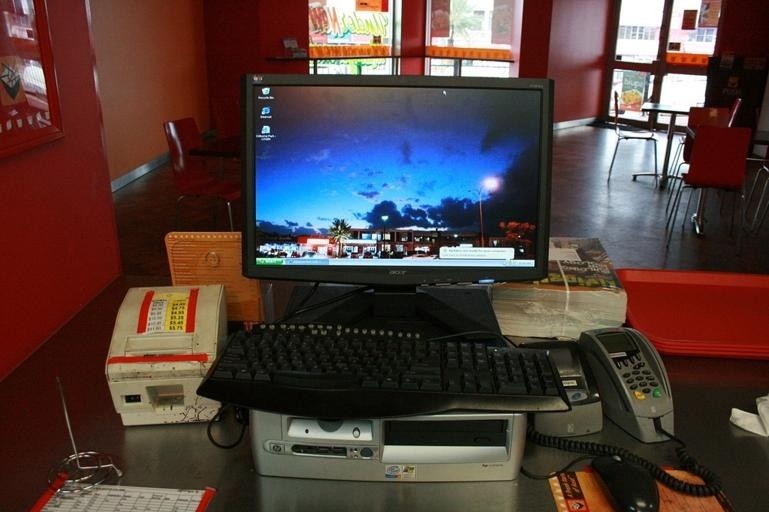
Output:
[103,284,229,428]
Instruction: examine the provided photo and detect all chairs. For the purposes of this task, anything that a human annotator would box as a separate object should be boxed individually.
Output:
[663,107,731,228]
[608,90,658,189]
[161,116,243,232]
[667,126,751,254]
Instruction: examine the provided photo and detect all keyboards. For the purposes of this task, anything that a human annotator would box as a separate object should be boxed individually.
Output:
[196,328,572,419]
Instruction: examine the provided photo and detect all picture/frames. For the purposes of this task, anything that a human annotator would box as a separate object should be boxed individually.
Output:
[0,0,65,158]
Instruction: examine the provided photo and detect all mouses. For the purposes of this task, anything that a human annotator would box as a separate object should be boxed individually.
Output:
[590,452,660,512]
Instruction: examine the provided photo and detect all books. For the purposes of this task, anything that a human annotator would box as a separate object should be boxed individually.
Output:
[492,235,628,337]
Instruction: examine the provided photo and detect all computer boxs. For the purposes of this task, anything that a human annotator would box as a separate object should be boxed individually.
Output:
[249,408,528,484]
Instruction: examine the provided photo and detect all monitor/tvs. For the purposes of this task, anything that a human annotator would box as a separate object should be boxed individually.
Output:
[240,71,555,326]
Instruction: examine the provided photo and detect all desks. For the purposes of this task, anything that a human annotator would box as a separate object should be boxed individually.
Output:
[1,272,769,512]
